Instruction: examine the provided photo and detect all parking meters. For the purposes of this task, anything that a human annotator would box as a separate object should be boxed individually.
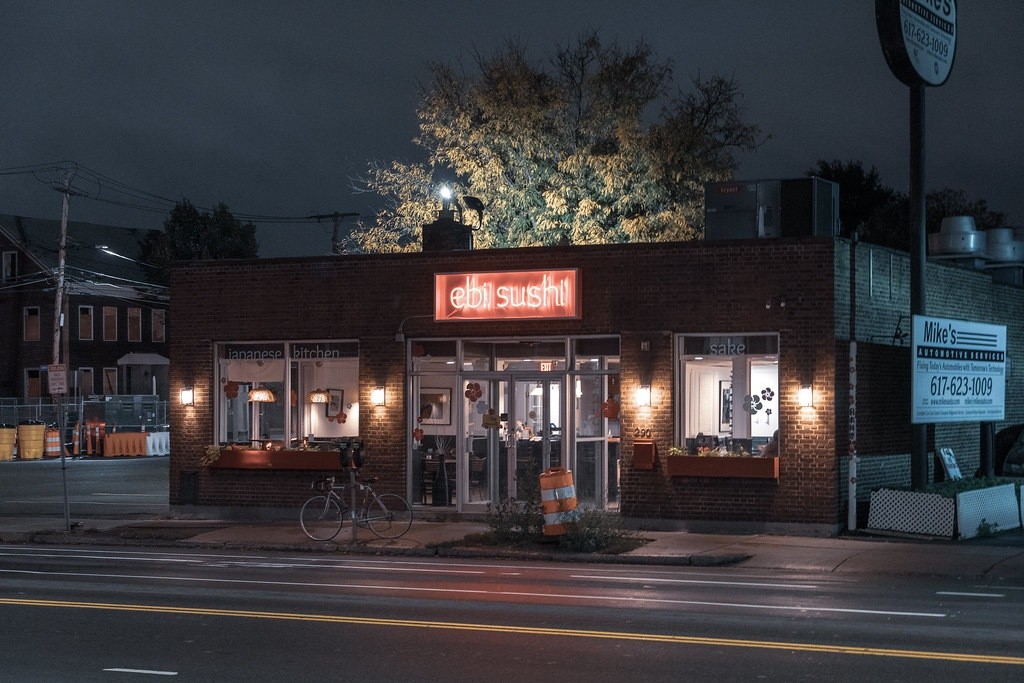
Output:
[337,437,364,544]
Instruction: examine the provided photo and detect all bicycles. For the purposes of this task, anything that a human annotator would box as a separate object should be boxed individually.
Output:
[299,473,413,541]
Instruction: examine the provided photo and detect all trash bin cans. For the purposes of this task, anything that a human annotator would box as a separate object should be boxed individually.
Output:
[18,419,46,459]
[0,423,17,460]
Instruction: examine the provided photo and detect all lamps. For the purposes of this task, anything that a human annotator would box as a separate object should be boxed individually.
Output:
[247,360,276,403]
[440,187,484,231]
[798,383,812,407]
[308,362,332,403]
[638,385,651,406]
[371,386,385,405]
[180,387,194,406]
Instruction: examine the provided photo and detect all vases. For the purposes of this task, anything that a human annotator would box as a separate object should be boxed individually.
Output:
[432,454,449,505]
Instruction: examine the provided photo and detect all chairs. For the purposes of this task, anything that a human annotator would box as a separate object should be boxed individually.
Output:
[449,460,485,504]
[422,459,440,503]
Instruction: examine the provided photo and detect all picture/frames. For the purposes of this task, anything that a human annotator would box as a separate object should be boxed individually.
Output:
[420,387,452,425]
[327,389,344,418]
